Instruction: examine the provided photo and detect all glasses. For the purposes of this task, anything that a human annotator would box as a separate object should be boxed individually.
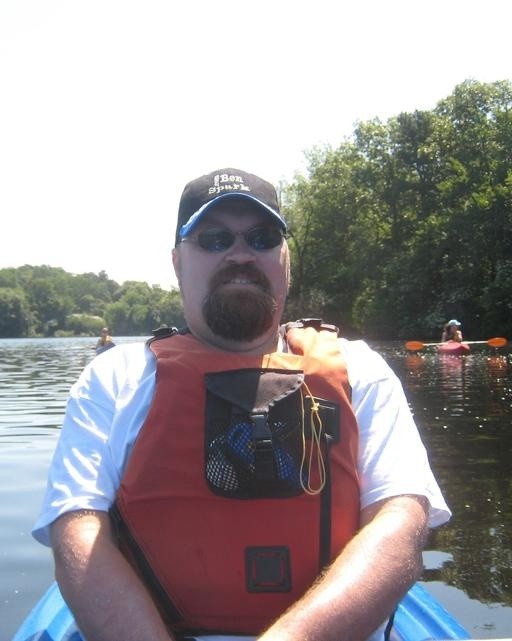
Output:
[180,224,281,248]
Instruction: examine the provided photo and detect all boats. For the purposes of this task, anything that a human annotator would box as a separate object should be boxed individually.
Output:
[95,341,115,355]
[13,572,470,641]
[437,343,470,354]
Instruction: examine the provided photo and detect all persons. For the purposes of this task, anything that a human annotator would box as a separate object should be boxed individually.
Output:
[95,326,112,349]
[31,168,454,641]
[442,319,462,341]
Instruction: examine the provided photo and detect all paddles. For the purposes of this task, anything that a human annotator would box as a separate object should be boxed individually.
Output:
[405,338,506,351]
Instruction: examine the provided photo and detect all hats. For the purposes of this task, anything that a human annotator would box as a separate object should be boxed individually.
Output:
[176,168,287,239]
[447,319,461,327]
